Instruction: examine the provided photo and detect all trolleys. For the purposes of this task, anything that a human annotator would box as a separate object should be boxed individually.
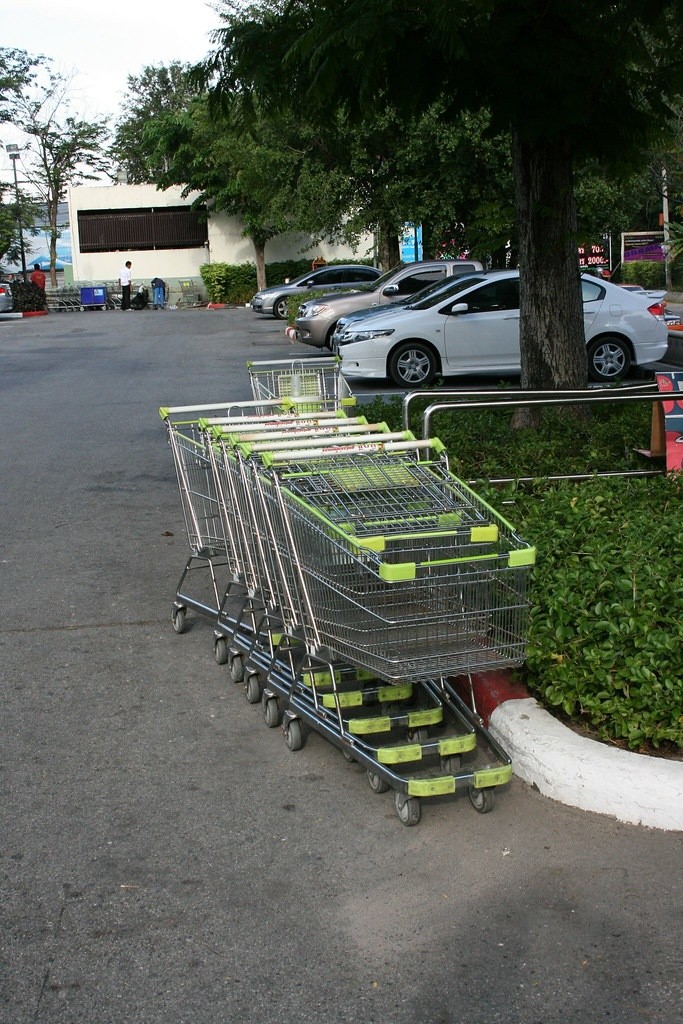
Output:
[155,393,536,829]
[47,279,169,312]
[243,356,355,420]
[176,278,204,308]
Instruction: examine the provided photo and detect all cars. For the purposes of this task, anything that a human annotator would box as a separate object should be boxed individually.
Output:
[620,284,682,334]
[250,265,382,321]
[0,283,13,314]
[335,270,669,384]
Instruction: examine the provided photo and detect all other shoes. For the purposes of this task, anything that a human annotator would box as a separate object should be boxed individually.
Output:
[152,305,164,310]
[120,309,134,312]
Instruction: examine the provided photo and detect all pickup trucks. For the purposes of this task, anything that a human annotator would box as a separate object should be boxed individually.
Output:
[294,259,485,352]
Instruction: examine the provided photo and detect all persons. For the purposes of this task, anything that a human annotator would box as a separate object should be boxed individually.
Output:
[151,278,165,310]
[30,264,46,291]
[5,274,14,283]
[120,261,134,312]
